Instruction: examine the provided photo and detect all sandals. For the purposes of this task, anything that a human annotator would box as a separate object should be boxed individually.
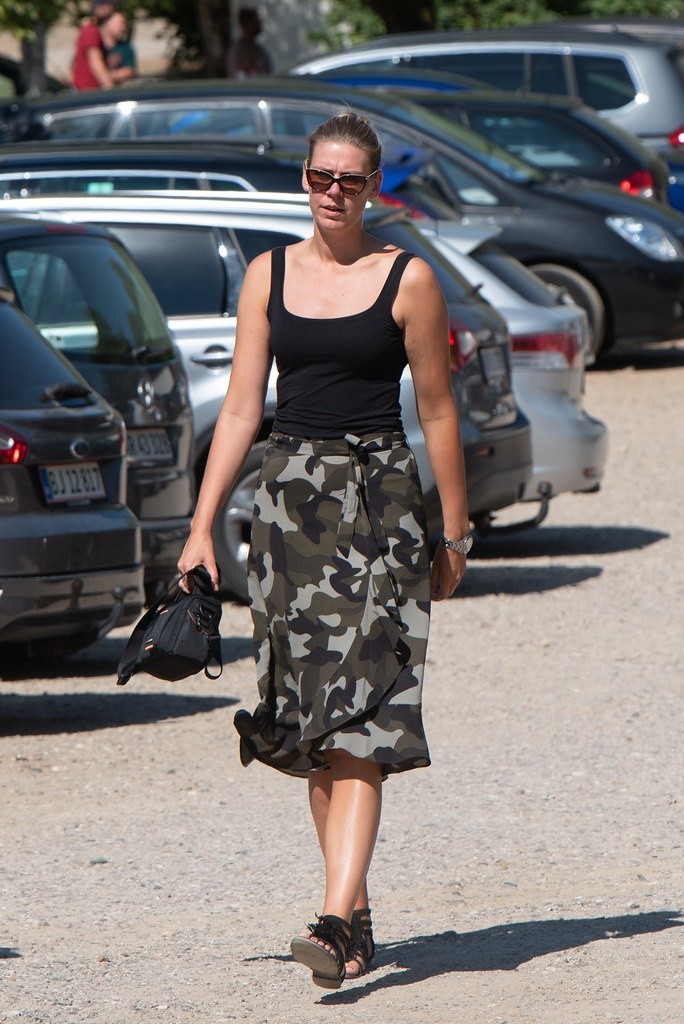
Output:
[343,909,376,977]
[290,915,351,988]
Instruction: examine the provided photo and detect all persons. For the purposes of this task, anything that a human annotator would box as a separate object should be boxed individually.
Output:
[226,8,275,78]
[71,0,140,91]
[176,110,474,989]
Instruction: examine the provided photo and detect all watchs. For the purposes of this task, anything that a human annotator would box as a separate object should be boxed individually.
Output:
[442,535,473,553]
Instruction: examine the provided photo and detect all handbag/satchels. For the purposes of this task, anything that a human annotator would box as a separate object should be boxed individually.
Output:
[117,561,223,684]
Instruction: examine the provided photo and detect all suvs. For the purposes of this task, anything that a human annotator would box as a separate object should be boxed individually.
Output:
[279,33,683,219]
[0,81,682,371]
[0,181,613,495]
[0,188,533,606]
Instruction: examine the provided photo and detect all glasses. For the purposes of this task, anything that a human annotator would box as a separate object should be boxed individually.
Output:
[305,159,378,195]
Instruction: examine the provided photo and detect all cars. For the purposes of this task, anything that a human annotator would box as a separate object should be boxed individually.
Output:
[0,218,194,607]
[0,297,143,658]
[380,88,671,210]
[1,138,451,235]
